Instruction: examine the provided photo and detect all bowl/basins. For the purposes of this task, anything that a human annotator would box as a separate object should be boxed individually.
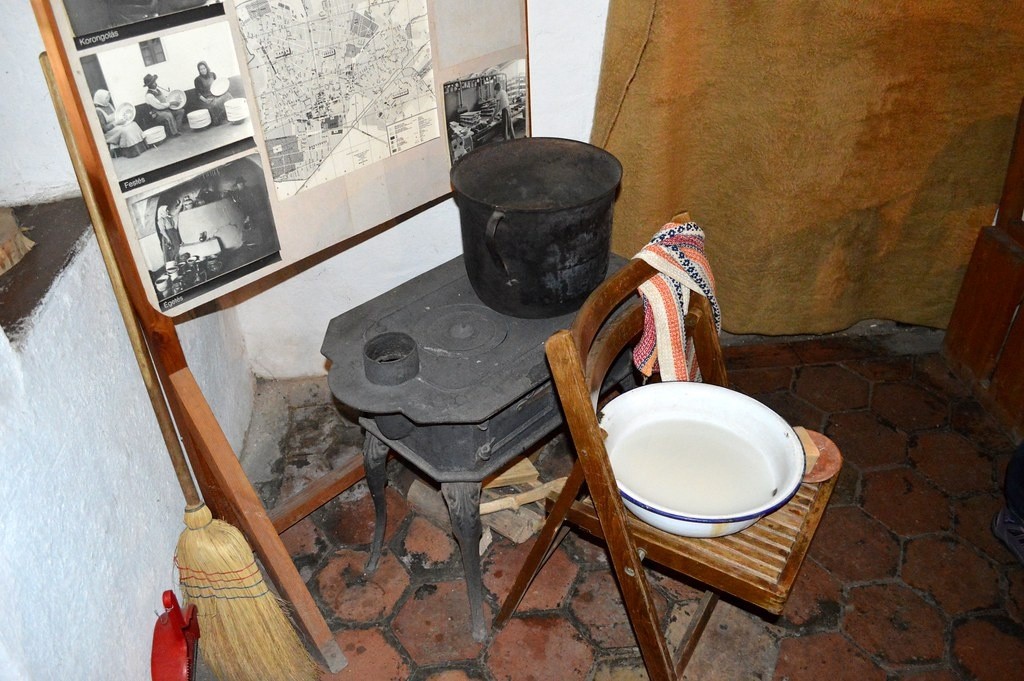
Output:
[595,381,804,537]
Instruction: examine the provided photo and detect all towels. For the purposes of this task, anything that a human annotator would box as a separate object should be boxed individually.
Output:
[631,210,724,386]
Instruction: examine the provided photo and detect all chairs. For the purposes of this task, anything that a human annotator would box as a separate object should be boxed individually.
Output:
[492,212,844,680]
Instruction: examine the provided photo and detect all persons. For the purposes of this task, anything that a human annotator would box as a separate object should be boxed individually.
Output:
[93,89,148,158]
[488,83,516,140]
[156,175,262,269]
[194,61,236,125]
[143,74,184,137]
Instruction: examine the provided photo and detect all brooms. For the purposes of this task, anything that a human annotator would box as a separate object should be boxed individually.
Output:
[40,54,324,681]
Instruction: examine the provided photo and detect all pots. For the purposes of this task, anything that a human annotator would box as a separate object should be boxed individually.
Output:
[451,138,622,315]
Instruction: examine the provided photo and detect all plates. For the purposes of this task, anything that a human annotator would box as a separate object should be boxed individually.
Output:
[789,428,842,482]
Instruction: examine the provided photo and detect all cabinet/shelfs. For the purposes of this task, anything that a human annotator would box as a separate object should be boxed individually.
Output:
[943,226,1023,435]
